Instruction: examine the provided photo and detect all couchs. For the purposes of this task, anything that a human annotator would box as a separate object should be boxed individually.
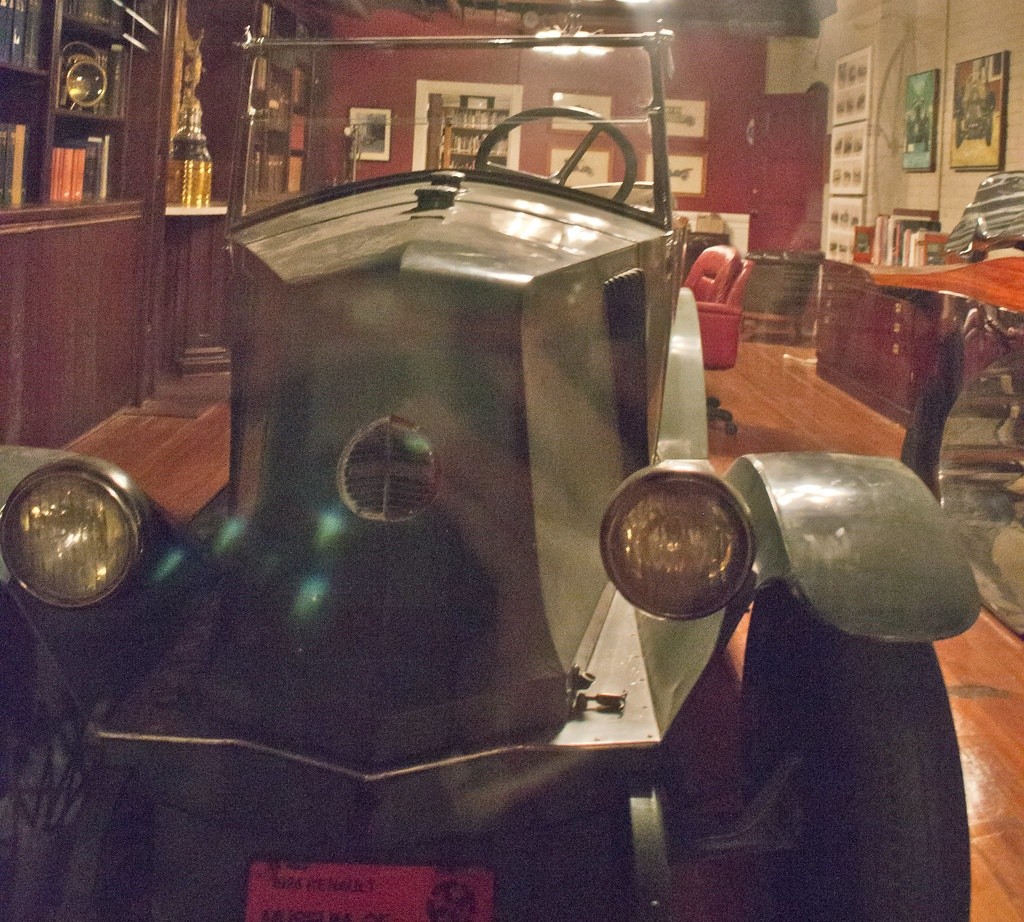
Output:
[685,245,753,371]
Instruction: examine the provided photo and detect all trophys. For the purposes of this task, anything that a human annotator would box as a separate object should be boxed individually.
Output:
[171,26,212,207]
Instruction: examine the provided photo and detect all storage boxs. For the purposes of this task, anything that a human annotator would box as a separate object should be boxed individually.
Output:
[696,216,724,235]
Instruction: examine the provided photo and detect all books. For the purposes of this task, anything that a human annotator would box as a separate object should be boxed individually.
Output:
[440,107,507,167]
[854,208,948,268]
[0,0,128,209]
[246,5,313,196]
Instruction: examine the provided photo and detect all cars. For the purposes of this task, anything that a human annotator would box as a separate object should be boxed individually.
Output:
[2,18,982,922]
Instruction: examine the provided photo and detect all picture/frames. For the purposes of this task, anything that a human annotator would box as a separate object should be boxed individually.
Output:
[902,68,940,172]
[644,96,711,142]
[550,89,614,138]
[644,150,709,197]
[949,50,1011,173]
[348,107,393,162]
[544,146,613,186]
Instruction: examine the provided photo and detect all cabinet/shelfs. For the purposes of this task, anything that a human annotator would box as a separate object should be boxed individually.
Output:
[0,1,321,450]
[814,258,999,433]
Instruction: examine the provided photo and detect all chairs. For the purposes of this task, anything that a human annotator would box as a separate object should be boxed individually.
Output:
[738,248,826,344]
[684,233,730,276]
